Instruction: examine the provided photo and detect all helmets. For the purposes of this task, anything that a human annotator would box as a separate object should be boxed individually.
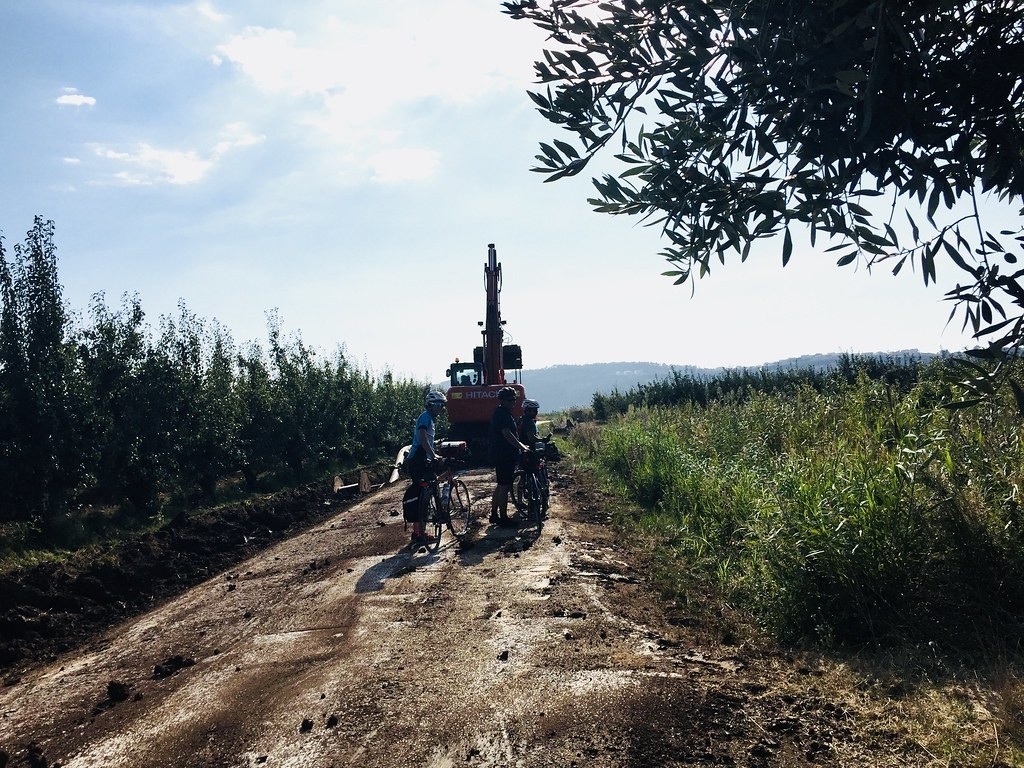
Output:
[523,401,539,408]
[496,387,516,400]
[425,391,447,406]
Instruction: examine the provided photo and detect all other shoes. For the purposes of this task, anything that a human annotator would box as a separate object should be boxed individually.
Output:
[411,533,434,544]
[501,517,518,527]
[489,516,499,523]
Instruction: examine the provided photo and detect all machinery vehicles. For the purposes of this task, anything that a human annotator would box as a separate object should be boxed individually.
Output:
[445,243,539,465]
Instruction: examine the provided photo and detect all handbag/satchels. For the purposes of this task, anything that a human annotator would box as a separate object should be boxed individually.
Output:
[402,485,428,531]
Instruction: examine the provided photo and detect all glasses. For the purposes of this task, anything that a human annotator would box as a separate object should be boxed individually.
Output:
[504,398,516,401]
[429,402,444,407]
[533,410,535,412]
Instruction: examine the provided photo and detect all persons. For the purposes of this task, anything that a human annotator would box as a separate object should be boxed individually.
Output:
[488,387,533,526]
[406,391,449,545]
[460,375,472,386]
[517,398,549,509]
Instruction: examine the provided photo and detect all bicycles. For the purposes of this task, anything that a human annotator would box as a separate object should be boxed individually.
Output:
[418,458,471,554]
[534,433,553,510]
[508,444,547,535]
